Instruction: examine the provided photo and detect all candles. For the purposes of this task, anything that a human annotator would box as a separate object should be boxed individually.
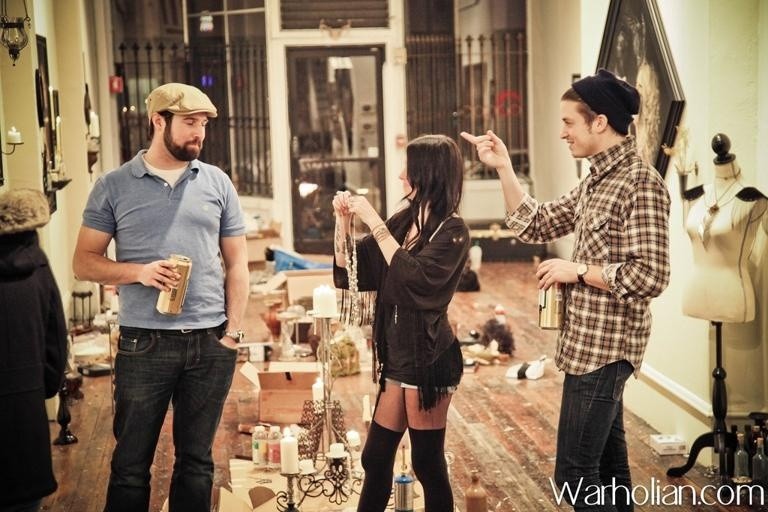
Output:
[48,85,56,130]
[91,111,101,136]
[311,281,337,319]
[299,374,371,476]
[56,116,63,162]
[279,428,301,474]
[7,126,22,145]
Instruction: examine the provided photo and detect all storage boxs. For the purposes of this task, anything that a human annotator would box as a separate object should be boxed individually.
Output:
[280,266,334,305]
[237,358,324,424]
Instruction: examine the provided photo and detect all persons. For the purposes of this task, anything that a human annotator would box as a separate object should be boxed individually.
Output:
[629,64,661,164]
[333,135,469,511]
[460,67,669,512]
[2,186,68,512]
[677,133,767,323]
[71,83,250,511]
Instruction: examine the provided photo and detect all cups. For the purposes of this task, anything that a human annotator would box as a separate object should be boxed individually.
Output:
[394,472,414,512]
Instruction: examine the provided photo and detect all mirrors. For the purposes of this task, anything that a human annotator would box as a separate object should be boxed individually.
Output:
[284,43,388,254]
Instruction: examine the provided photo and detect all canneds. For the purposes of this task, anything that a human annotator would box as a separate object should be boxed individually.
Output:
[155,253,192,315]
[537,277,565,330]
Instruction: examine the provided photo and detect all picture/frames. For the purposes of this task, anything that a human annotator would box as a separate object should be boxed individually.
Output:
[593,0,687,185]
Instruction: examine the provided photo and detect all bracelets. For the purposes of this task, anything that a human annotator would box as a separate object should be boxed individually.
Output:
[370,221,389,242]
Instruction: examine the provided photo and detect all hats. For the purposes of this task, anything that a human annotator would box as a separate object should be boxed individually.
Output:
[570,68,642,137]
[143,81,218,127]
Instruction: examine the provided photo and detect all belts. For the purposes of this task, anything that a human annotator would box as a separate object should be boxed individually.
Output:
[118,320,228,336]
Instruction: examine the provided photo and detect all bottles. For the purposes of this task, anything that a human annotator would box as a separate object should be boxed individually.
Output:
[727,423,766,491]
[493,302,507,326]
[470,239,483,274]
[250,422,281,470]
[102,283,116,309]
[464,475,487,511]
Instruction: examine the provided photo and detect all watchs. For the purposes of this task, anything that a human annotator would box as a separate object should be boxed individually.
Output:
[576,263,588,287]
[223,328,245,344]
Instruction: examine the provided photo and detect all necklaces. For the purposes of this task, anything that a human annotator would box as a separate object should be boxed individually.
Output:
[342,208,359,326]
